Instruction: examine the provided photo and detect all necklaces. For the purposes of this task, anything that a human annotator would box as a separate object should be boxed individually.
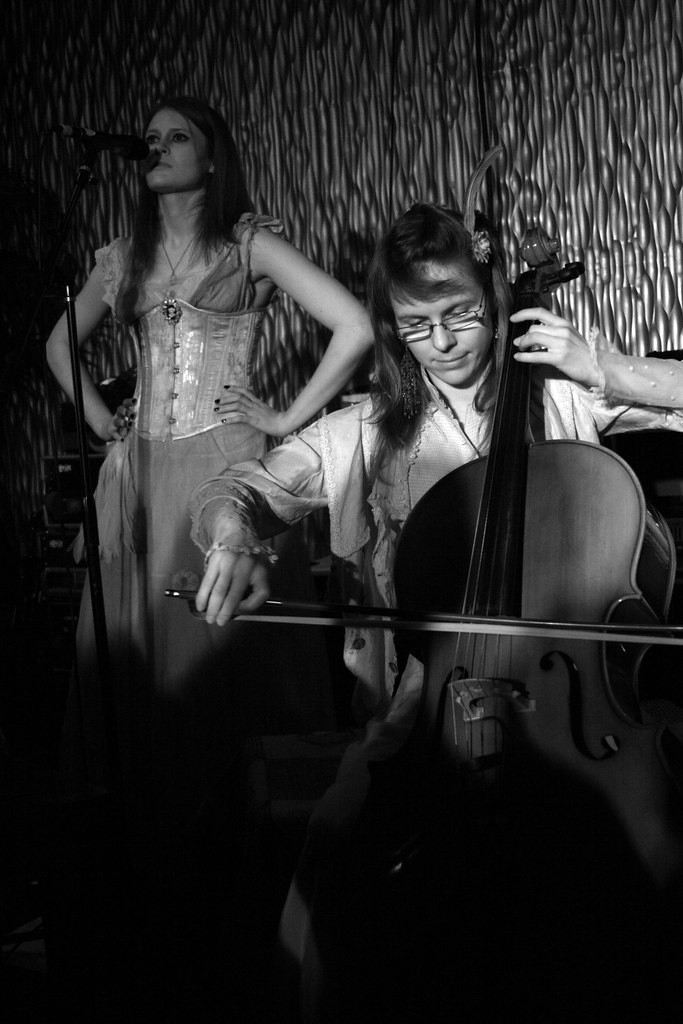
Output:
[160,226,203,279]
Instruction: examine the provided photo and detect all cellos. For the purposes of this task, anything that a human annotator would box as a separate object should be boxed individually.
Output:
[280,231,683,1024]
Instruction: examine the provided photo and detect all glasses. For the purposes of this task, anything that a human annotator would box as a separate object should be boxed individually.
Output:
[393,285,487,343]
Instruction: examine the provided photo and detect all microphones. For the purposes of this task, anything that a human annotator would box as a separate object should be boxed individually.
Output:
[53,124,150,160]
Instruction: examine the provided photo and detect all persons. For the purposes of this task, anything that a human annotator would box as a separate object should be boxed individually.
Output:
[191,205,683,1024]
[44,96,376,830]
